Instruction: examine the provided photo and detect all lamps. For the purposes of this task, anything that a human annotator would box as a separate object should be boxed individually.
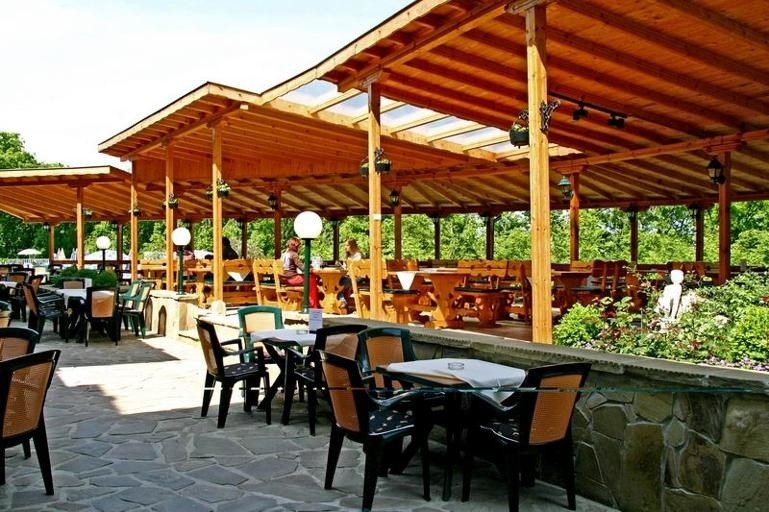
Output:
[558,176,574,202]
[267,192,280,210]
[569,102,625,130]
[705,156,728,187]
[388,186,401,206]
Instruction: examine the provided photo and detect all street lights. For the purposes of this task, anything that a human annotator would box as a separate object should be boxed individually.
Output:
[172,224,191,295]
[95,236,113,270]
[292,210,323,313]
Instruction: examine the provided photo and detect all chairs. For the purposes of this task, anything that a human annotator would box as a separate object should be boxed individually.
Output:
[316,348,434,511]
[461,361,593,512]
[0,325,41,461]
[138,254,712,329]
[0,262,155,347]
[0,348,64,498]
[357,326,445,478]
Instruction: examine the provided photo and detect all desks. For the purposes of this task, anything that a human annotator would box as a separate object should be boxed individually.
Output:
[374,357,526,502]
[249,325,317,412]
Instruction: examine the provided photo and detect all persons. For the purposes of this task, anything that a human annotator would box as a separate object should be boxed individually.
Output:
[183,245,196,280]
[281,236,320,308]
[204,237,241,289]
[336,239,362,308]
[173,245,182,261]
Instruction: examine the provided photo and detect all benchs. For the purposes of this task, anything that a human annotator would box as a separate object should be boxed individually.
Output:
[236,306,298,407]
[279,324,368,437]
[191,316,273,429]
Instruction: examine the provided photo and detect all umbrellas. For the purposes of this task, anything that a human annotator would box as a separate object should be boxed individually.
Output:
[18,248,42,263]
